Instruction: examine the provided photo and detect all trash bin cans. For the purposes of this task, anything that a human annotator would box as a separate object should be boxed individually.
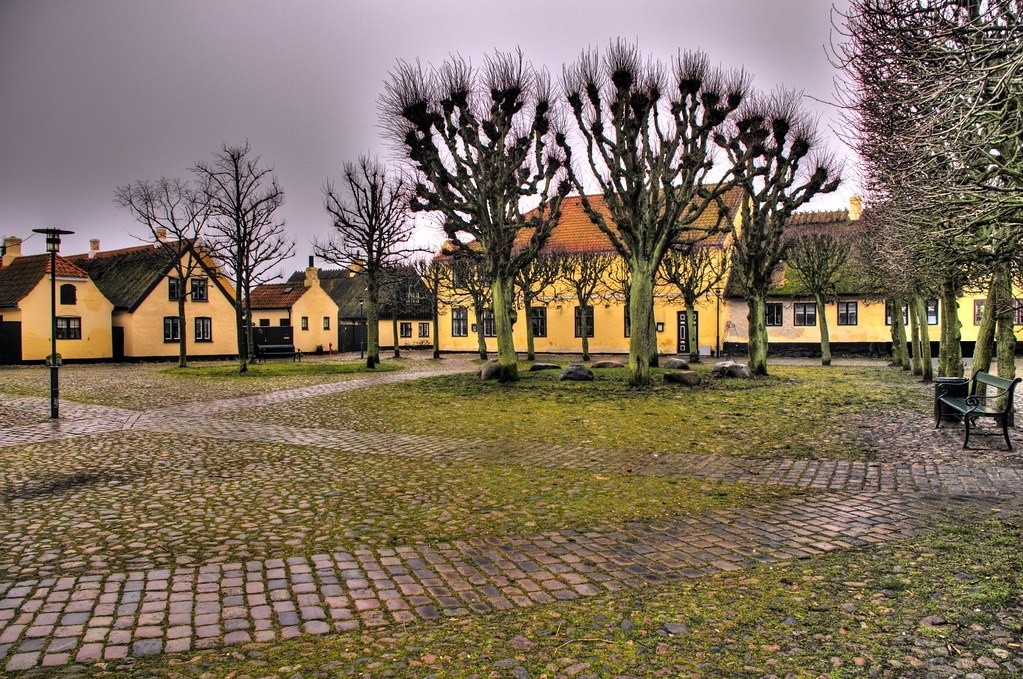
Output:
[934,377,969,423]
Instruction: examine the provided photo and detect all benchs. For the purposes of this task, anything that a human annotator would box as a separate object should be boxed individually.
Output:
[257,343,302,363]
[934,369,1023,451]
